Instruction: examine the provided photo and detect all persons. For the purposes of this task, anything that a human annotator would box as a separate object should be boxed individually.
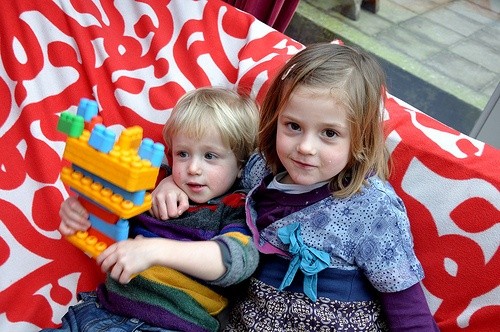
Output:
[37,87,260,332]
[147,43,442,332]
